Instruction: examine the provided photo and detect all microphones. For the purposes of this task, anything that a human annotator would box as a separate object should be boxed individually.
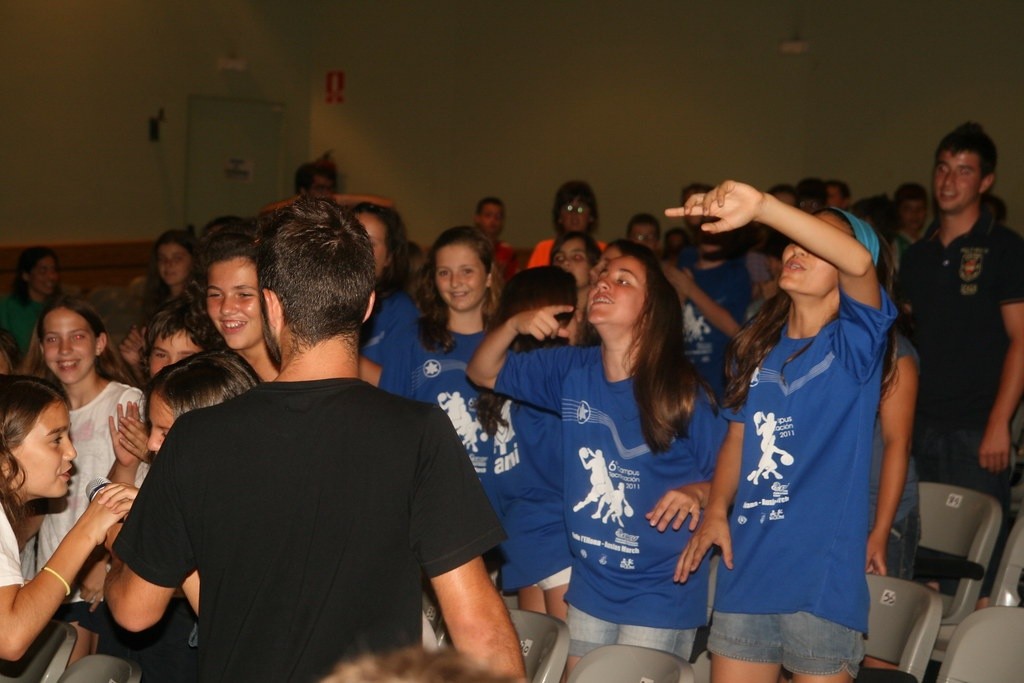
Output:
[86,477,124,524]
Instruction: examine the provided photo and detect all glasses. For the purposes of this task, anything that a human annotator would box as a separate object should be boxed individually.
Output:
[632,231,656,241]
[565,204,583,214]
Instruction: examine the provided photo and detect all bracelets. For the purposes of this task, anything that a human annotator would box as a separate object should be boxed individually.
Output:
[41,566,71,595]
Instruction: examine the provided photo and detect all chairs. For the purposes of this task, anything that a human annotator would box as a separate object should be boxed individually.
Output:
[0,484,1024,683]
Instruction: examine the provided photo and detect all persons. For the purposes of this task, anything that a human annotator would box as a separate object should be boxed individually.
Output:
[0,119,1024,683]
